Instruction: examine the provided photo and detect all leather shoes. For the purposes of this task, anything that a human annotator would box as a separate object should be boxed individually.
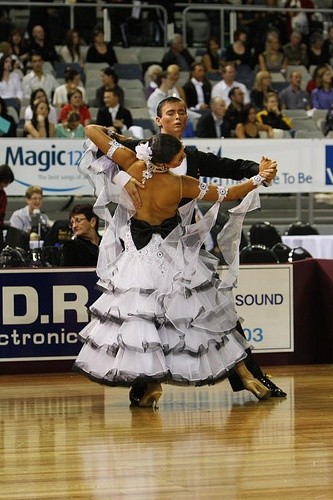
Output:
[129,380,147,406]
[257,376,287,397]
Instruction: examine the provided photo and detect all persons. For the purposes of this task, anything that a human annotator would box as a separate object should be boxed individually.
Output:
[235,103,274,138]
[60,204,103,268]
[145,1,333,138]
[96,96,287,404]
[84,125,277,409]
[9,186,51,232]
[0,26,133,139]
[196,96,231,138]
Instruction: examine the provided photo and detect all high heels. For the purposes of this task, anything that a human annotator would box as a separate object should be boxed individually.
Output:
[241,376,271,400]
[138,382,163,409]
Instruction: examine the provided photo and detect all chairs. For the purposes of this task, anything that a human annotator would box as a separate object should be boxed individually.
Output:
[0,0,333,267]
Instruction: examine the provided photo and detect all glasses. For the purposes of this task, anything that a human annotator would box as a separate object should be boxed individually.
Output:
[68,217,88,228]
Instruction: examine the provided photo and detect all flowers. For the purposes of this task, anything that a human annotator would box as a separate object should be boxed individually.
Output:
[135,141,153,165]
